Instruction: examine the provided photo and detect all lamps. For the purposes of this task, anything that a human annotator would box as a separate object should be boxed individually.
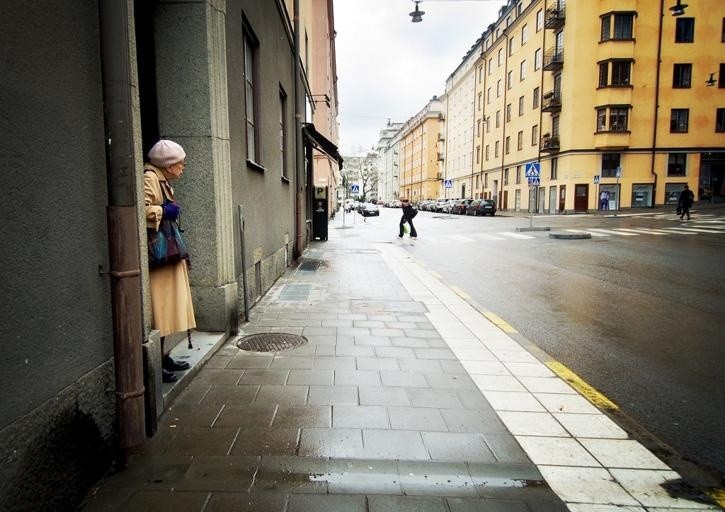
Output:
[669,0,688,16]
[704,69,723,86]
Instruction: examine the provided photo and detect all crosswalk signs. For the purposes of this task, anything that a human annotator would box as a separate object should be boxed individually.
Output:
[351,185,360,194]
[445,180,452,188]
[524,163,541,177]
[594,176,599,184]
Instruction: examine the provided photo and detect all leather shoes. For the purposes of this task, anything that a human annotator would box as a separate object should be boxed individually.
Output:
[162,354,189,383]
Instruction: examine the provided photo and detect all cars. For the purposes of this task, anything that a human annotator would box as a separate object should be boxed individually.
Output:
[362,204,379,217]
[372,198,496,216]
[358,205,362,214]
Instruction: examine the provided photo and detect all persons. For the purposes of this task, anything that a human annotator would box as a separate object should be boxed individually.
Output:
[678,185,694,220]
[600,188,609,210]
[345,200,349,214]
[399,197,417,238]
[143,140,196,383]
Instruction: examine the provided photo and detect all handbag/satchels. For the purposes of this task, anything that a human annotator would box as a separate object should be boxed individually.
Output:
[146,219,190,266]
[411,207,418,218]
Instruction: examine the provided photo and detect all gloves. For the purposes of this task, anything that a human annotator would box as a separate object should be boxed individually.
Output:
[163,202,182,220]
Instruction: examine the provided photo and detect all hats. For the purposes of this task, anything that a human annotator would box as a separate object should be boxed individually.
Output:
[148,139,186,168]
